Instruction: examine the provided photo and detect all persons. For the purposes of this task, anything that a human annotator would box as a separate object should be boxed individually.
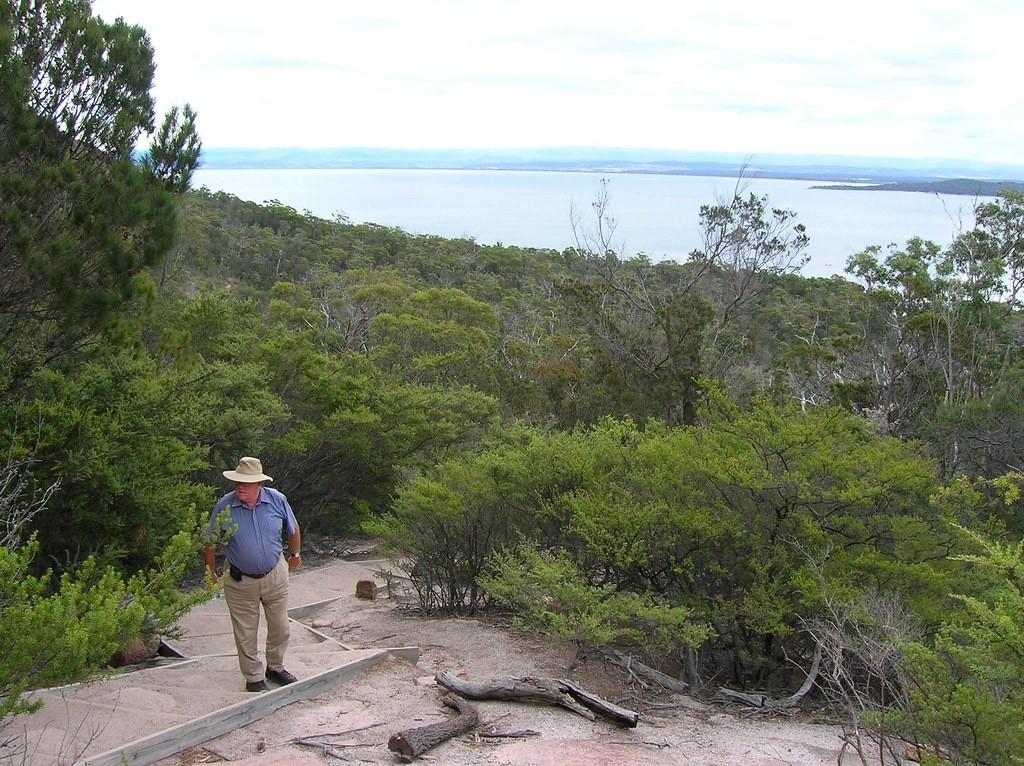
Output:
[205,457,301,692]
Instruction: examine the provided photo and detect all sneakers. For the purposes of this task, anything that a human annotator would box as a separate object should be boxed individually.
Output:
[246,679,270,693]
[265,666,297,685]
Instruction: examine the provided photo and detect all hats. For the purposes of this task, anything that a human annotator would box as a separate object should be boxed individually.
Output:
[222,457,273,483]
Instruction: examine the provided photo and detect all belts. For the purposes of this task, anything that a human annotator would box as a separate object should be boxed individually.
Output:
[241,569,272,580]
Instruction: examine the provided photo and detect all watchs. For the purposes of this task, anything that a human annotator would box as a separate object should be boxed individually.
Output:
[291,553,301,558]
